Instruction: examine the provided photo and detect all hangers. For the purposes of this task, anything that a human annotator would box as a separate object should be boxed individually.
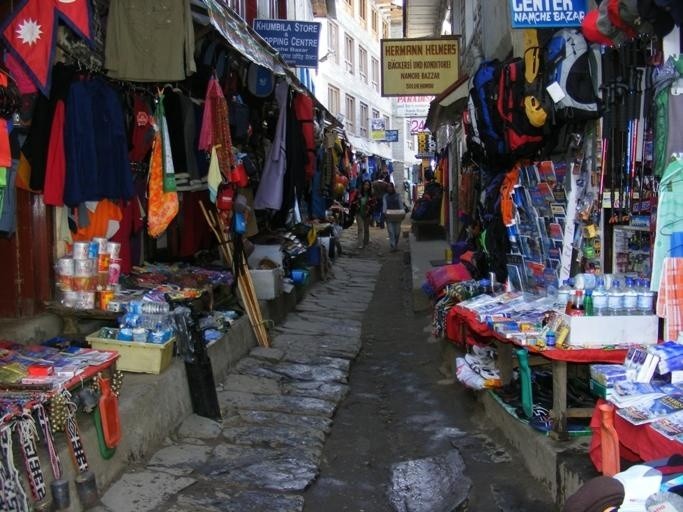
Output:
[68,57,160,101]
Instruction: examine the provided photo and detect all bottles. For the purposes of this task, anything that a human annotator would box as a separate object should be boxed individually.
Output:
[557,270,654,315]
[112,298,173,343]
[541,311,556,346]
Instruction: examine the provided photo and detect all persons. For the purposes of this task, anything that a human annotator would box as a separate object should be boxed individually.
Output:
[380,182,405,252]
[350,180,378,249]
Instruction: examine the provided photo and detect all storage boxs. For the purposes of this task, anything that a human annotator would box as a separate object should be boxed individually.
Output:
[85,327,177,376]
[247,268,281,300]
[386,209,406,223]
[550,308,661,349]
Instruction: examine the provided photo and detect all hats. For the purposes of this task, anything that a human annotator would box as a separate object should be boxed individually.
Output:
[582,0,681,45]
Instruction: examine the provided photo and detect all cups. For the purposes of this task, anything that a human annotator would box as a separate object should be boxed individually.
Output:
[479,278,492,293]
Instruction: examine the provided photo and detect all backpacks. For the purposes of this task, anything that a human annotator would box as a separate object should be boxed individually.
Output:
[461,29,600,167]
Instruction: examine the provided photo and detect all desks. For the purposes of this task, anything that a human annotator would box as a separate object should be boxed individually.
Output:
[590,398,683,466]
[459,293,670,441]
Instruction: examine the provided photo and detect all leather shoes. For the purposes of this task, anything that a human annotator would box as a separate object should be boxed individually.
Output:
[493,378,594,432]
